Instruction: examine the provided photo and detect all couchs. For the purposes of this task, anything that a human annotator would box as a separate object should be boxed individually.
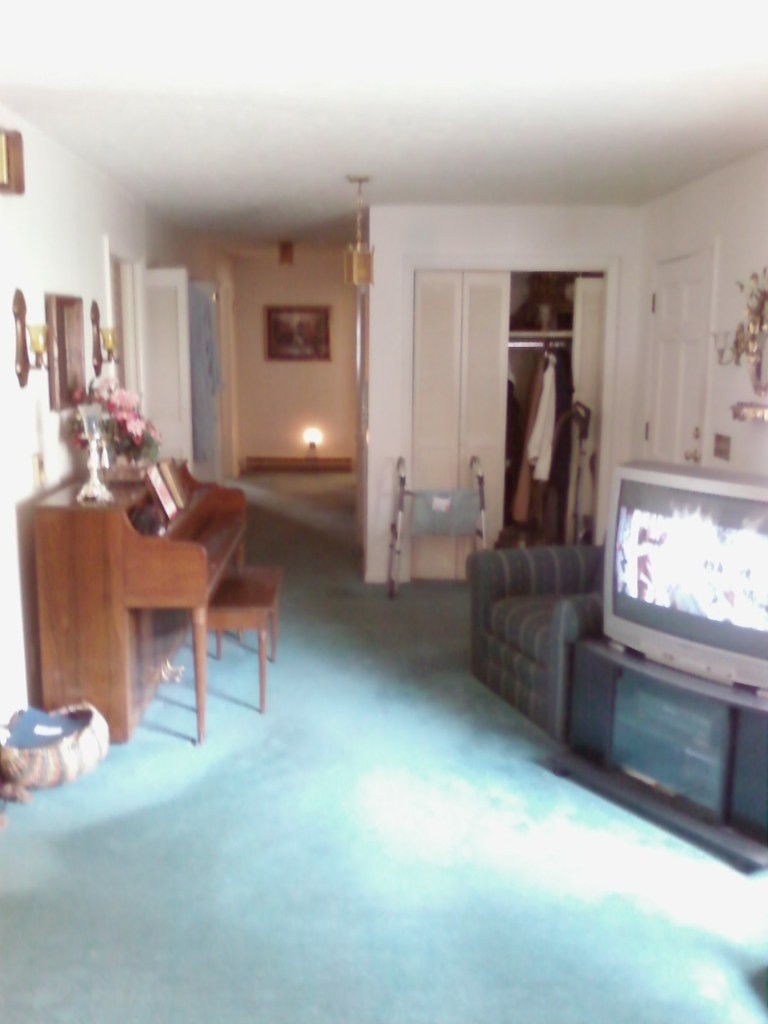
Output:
[462,545,606,741]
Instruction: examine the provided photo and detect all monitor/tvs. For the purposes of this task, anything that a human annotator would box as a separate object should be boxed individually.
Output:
[603,460,768,703]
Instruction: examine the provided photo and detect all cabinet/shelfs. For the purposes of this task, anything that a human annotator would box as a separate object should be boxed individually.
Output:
[531,642,768,876]
[410,270,606,582]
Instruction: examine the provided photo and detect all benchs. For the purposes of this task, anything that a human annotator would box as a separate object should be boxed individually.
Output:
[207,572,277,713]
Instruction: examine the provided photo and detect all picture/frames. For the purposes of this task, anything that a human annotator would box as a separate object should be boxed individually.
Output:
[262,304,332,361]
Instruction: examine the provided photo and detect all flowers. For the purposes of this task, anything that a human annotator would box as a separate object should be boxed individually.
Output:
[74,380,160,468]
